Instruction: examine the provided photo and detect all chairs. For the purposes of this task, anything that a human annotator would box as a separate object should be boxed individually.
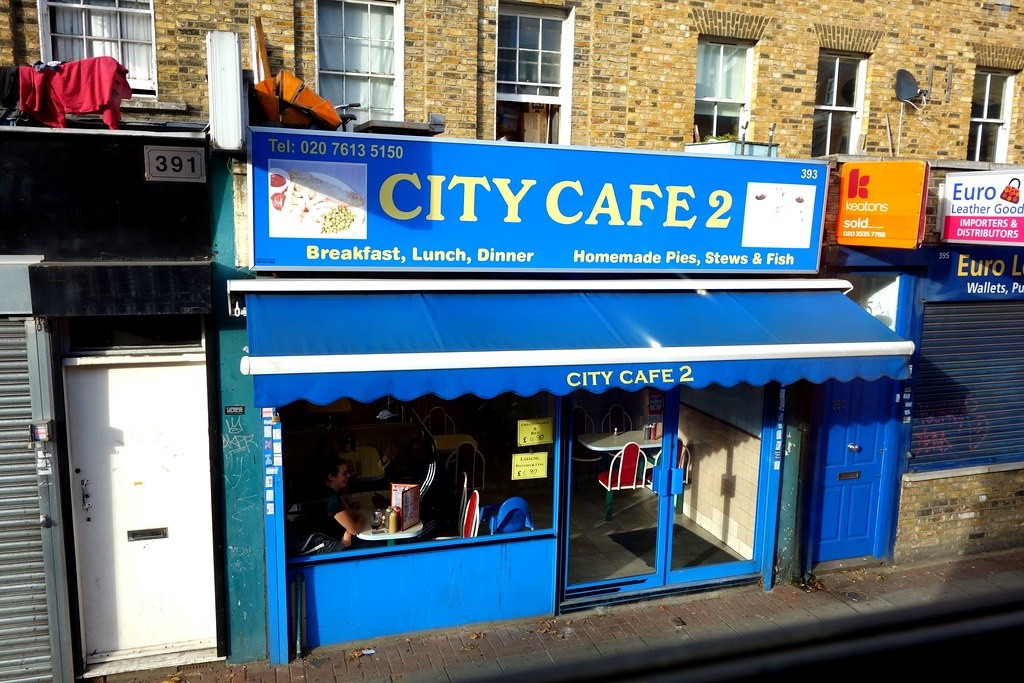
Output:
[430,470,480,540]
[479,497,534,536]
[382,442,398,468]
[601,404,642,459]
[597,444,647,522]
[444,441,485,496]
[421,407,456,437]
[571,405,602,480]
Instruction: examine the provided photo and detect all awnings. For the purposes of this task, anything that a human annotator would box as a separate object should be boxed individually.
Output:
[240,291,916,408]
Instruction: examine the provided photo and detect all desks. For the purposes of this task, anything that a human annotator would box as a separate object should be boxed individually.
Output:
[350,491,423,544]
[578,430,662,451]
[338,446,385,481]
[353,119,445,137]
[433,434,478,452]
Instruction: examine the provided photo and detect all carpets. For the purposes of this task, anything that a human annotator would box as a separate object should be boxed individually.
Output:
[606,524,739,570]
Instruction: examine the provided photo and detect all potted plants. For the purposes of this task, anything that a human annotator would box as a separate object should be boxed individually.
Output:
[684,133,780,157]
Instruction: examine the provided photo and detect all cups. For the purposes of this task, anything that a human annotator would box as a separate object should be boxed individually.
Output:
[370,517,381,530]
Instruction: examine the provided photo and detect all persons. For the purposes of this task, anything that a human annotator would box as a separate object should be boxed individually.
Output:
[401,442,447,544]
[312,456,381,550]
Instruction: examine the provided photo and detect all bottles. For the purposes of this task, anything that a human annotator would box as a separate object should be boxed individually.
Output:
[614,427,617,436]
[373,510,382,526]
[643,423,656,439]
[385,505,401,534]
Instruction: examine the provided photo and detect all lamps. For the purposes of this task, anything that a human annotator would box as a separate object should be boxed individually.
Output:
[308,399,353,432]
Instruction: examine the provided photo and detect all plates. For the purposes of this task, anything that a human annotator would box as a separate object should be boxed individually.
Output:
[285,172,365,238]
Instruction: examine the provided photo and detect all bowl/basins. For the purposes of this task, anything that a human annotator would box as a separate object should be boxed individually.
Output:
[269,168,290,193]
